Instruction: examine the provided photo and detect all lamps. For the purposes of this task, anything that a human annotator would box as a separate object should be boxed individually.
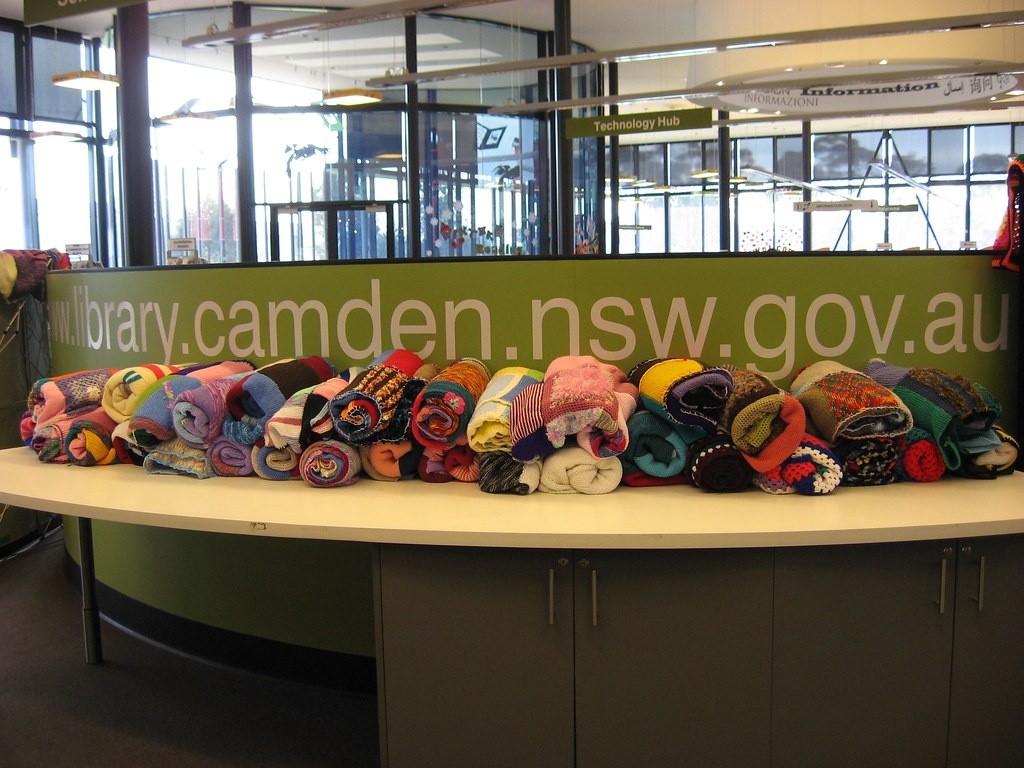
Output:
[51,69,119,91]
[322,88,382,107]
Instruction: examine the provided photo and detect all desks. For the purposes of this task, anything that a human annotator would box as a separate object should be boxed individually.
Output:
[1,447,1024,668]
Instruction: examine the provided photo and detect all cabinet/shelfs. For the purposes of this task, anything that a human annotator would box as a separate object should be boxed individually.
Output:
[371,547,777,768]
[775,531,1024,768]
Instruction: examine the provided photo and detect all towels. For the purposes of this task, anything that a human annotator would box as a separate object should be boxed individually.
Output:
[17,348,1021,497]
